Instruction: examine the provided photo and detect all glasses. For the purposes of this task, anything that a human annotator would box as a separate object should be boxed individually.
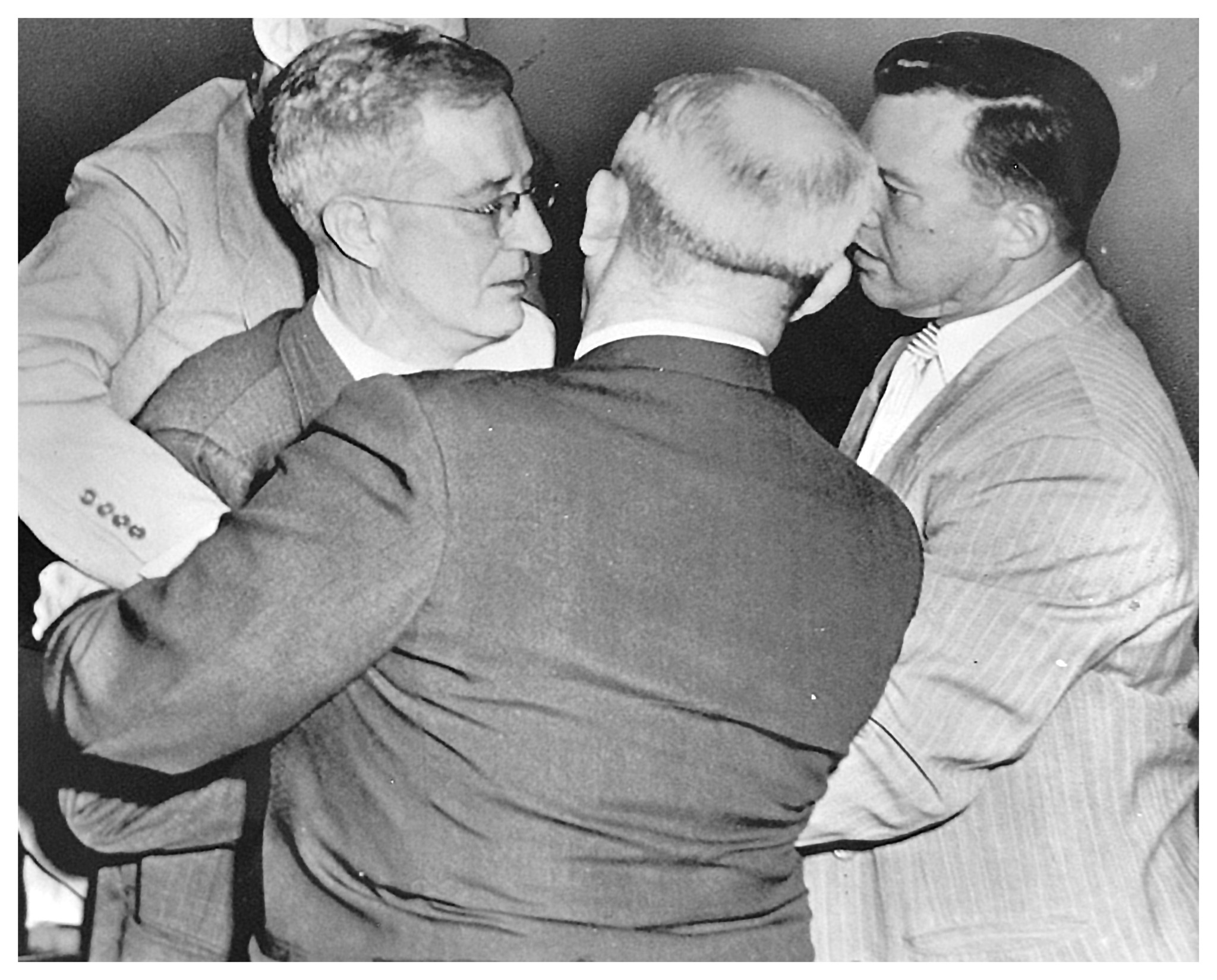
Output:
[351,177,558,223]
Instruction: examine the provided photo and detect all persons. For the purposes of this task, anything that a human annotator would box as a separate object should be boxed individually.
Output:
[795,32,1197,964]
[31,63,924,964]
[18,20,467,961]
[56,32,551,962]
